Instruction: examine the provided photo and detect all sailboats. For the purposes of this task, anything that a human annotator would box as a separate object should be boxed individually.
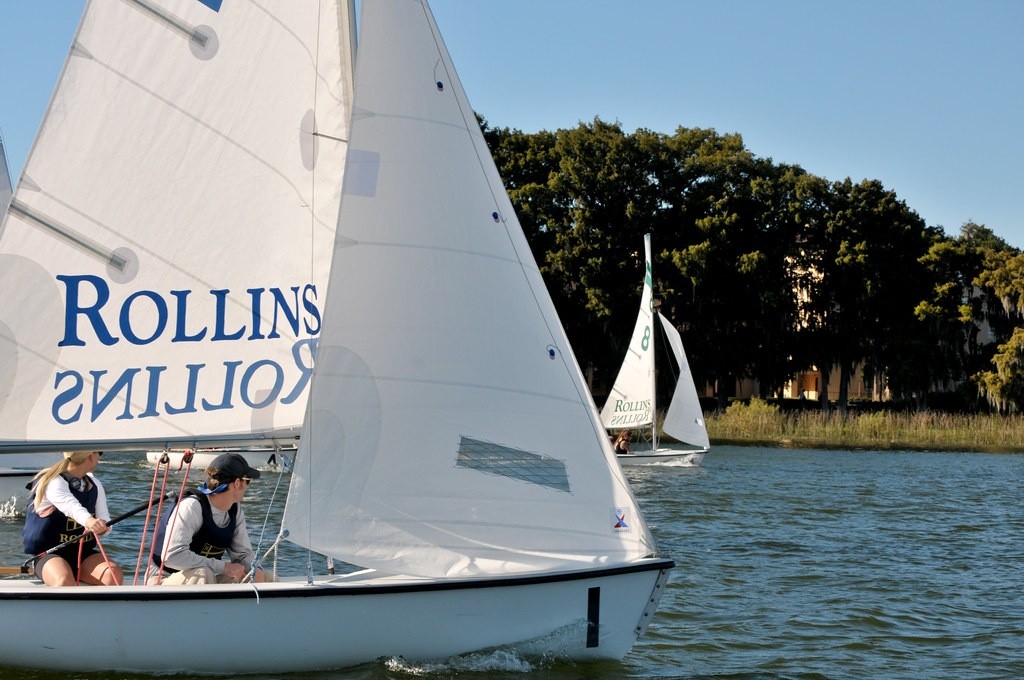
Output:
[592,233,710,466]
[0,0,676,674]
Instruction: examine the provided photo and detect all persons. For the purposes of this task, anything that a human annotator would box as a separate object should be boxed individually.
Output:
[614,429,633,454]
[143,452,278,586]
[25,450,124,587]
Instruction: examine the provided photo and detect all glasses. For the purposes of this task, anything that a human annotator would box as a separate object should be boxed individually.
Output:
[241,477,251,484]
[94,452,103,456]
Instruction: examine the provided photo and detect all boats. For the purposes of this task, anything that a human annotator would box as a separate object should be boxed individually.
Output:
[145,444,297,475]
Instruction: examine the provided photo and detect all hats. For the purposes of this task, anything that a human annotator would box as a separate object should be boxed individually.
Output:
[209,453,261,482]
